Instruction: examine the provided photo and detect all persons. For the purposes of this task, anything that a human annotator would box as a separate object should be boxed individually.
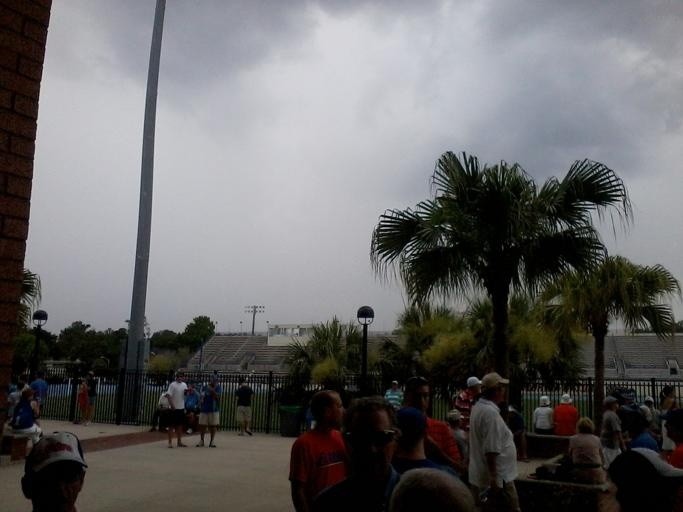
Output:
[286,369,683,512]
[19,430,89,512]
[77,377,91,423]
[78,370,98,426]
[147,371,256,448]
[0,369,50,446]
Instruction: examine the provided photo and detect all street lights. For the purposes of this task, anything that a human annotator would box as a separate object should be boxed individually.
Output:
[215,321,218,336]
[614,315,618,334]
[265,320,269,328]
[31,309,47,380]
[356,305,374,396]
[239,320,242,336]
[243,304,264,336]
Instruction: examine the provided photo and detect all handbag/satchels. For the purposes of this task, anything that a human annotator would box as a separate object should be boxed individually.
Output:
[477,483,512,511]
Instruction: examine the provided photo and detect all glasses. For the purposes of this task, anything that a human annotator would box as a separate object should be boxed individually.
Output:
[348,430,396,449]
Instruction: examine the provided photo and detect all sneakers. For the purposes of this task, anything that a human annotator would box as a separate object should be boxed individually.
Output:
[150,424,218,448]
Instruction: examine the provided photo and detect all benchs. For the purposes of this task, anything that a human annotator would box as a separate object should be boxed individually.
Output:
[525,432,580,459]
[1,432,30,462]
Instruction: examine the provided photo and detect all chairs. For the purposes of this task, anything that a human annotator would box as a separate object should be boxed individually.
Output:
[607,447,683,512]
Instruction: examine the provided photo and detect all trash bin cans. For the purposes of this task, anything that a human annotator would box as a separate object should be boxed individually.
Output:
[280,406,302,437]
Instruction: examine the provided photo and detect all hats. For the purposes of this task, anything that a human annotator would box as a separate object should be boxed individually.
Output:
[659,407,683,426]
[392,407,426,441]
[539,395,553,408]
[558,393,575,405]
[24,431,87,476]
[602,394,618,407]
[622,390,636,401]
[645,394,655,404]
[481,373,510,387]
[446,408,465,421]
[467,375,483,386]
